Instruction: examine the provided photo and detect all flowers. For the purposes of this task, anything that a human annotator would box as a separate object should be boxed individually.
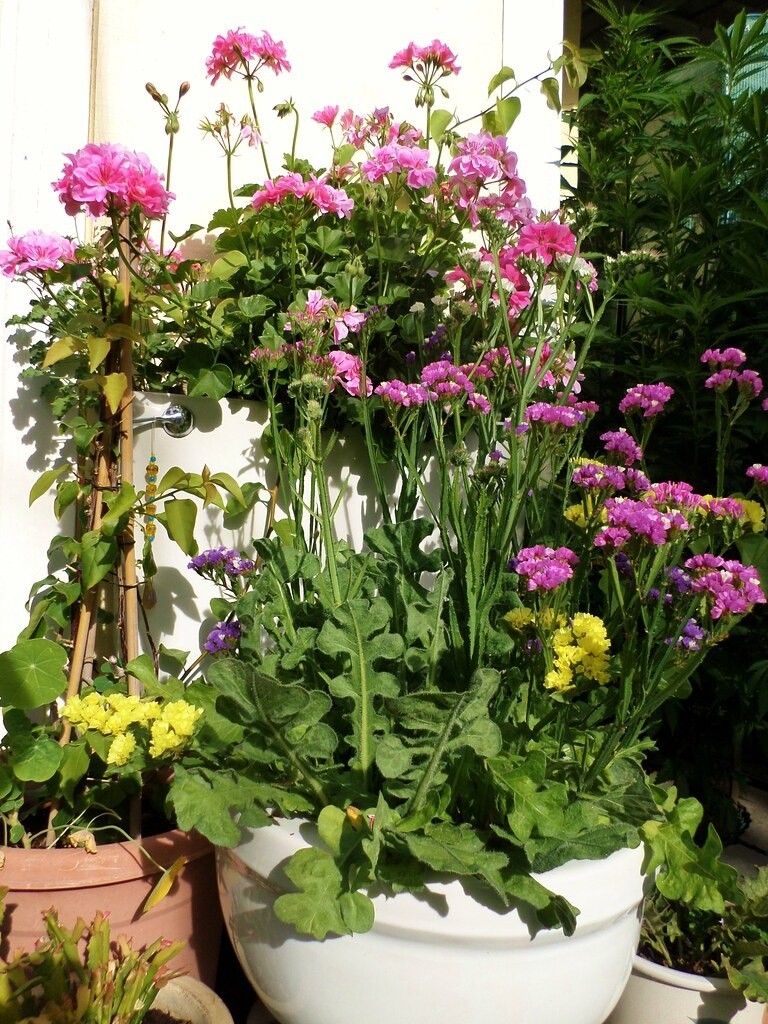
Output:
[0,26,768,948]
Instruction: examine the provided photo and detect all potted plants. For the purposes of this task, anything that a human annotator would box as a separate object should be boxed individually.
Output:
[0,905,235,1024]
[607,863,768,1024]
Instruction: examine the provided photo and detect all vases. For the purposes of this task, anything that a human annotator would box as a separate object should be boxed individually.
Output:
[216,808,664,1024]
[0,769,222,987]
